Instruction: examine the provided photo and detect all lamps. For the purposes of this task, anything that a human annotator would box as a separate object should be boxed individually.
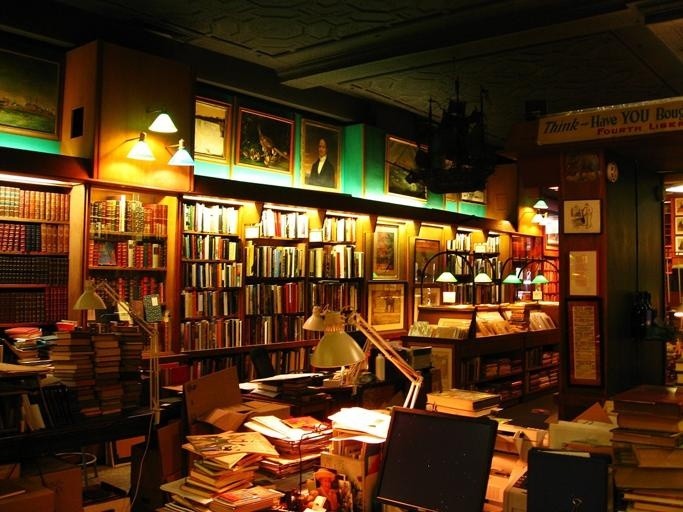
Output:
[167,139,199,167]
[530,273,549,284]
[435,272,458,283]
[302,307,324,332]
[127,131,154,161]
[309,313,367,368]
[532,199,549,209]
[475,272,492,283]
[674,303,683,318]
[148,106,179,134]
[74,279,106,310]
[502,273,522,285]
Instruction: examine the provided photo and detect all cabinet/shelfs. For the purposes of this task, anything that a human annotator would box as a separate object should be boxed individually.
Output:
[181,192,372,378]
[372,201,511,332]
[0,169,180,357]
[405,297,560,408]
[513,232,549,300]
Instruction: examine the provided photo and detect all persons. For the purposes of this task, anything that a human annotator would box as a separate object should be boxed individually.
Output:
[309,137,336,188]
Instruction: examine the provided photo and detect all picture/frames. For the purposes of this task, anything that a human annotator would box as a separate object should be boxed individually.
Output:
[193,96,234,165]
[565,295,604,389]
[0,46,67,141]
[366,279,409,332]
[235,106,296,174]
[413,238,441,285]
[459,183,489,206]
[299,118,342,193]
[411,285,443,324]
[384,132,430,201]
[558,197,604,237]
[372,223,400,279]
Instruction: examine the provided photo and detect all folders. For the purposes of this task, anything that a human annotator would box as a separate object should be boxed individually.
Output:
[526,444,610,512]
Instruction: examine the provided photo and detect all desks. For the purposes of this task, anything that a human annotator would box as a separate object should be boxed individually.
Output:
[0,393,185,465]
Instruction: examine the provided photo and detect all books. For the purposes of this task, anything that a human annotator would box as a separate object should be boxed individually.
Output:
[0,185,71,222]
[181,233,240,261]
[542,258,560,301]
[261,208,309,240]
[406,317,472,339]
[245,316,305,345]
[0,223,70,253]
[528,366,561,394]
[244,280,305,315]
[250,372,332,398]
[525,346,561,369]
[309,244,365,279]
[88,236,165,268]
[180,289,238,319]
[0,485,27,500]
[182,202,239,234]
[458,356,523,383]
[0,255,69,285]
[158,414,386,512]
[88,274,166,322]
[425,381,683,512]
[0,286,69,325]
[322,216,357,242]
[0,324,146,433]
[244,240,306,278]
[475,302,557,340]
[181,262,243,288]
[309,280,360,339]
[191,347,308,383]
[89,194,168,235]
[180,318,243,353]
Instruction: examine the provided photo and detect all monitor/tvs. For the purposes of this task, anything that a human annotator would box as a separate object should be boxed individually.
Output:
[373,407,498,511]
